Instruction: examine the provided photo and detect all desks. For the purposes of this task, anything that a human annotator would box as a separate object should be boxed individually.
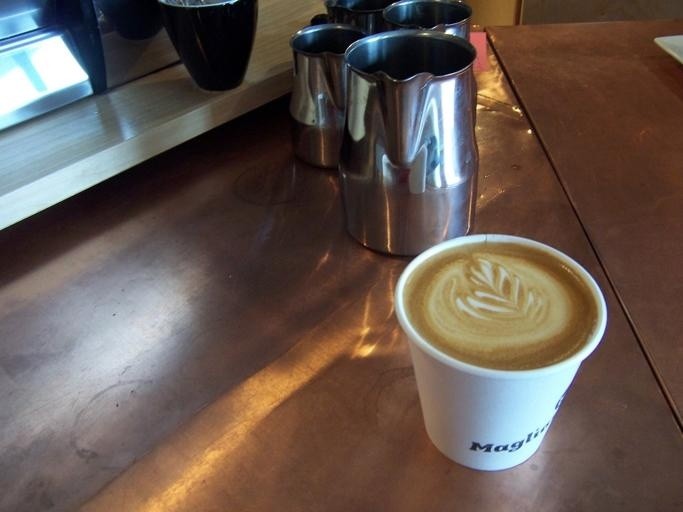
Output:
[0,29,683,511]
[485,19,683,429]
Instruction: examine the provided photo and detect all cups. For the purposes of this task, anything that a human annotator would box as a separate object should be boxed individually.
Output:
[97,0,163,43]
[323,1,386,37]
[162,0,260,91]
[394,231,608,468]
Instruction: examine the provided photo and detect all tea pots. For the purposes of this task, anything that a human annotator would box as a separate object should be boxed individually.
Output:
[339,30,480,251]
[383,2,473,39]
[283,25,358,170]
